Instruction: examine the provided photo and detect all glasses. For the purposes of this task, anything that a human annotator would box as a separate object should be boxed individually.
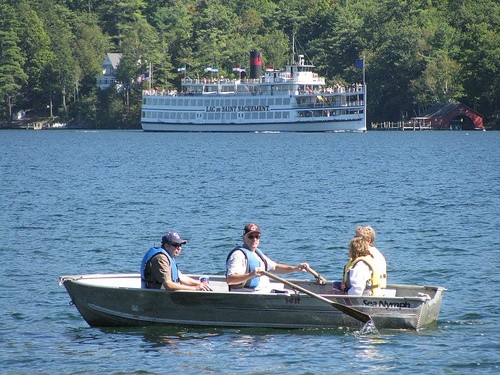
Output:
[169,244,181,247]
[246,235,261,239]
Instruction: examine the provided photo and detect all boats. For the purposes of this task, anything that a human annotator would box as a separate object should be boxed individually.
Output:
[140,33,369,132]
[59,274,448,330]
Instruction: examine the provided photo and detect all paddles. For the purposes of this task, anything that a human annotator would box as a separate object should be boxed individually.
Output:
[299,264,326,280]
[260,270,372,324]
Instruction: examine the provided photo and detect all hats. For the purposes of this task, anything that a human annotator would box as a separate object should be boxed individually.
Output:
[162,232,186,244]
[244,224,261,236]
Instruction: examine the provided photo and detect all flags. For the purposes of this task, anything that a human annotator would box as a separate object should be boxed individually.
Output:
[213,67,218,73]
[232,65,239,72]
[137,72,149,83]
[178,66,186,72]
[204,66,212,74]
[240,66,245,71]
[356,60,363,70]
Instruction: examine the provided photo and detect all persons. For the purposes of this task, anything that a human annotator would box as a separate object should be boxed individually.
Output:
[324,237,381,296]
[354,226,386,288]
[225,223,309,294]
[141,232,213,291]
